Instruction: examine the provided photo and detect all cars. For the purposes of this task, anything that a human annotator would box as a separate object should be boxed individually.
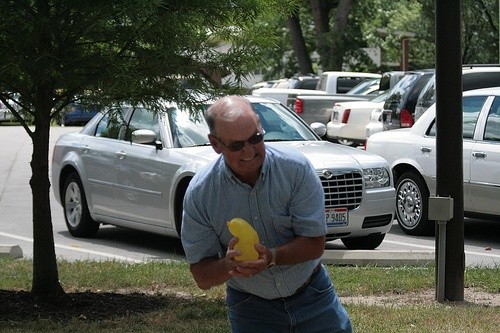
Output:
[51,92,395,250]
[0,92,35,121]
[249,64,500,236]
[56,96,103,126]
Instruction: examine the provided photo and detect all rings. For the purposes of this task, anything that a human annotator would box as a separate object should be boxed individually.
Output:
[249,274,252,279]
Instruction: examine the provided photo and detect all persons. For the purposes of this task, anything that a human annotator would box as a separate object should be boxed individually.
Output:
[181,94,354,333]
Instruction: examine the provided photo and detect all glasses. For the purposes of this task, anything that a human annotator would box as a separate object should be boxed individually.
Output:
[215,123,265,152]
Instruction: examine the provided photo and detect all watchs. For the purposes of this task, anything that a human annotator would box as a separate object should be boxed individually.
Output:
[268,248,275,270]
